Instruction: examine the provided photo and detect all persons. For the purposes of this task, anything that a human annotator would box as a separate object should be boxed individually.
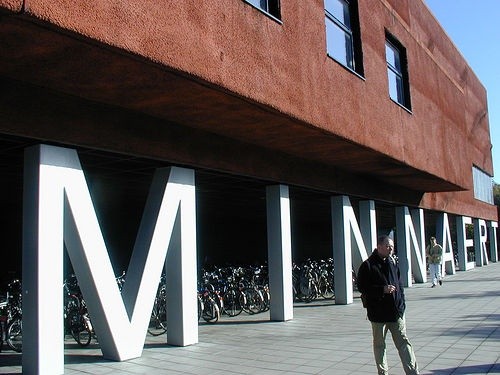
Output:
[357,235,421,375]
[426,236,443,288]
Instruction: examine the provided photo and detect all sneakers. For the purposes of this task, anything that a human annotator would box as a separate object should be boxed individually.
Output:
[438,280,442,286]
[431,283,436,288]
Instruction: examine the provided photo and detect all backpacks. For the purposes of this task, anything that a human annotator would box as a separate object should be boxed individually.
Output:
[360,256,388,308]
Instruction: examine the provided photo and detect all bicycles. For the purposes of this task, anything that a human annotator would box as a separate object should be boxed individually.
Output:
[0,257,357,352]
